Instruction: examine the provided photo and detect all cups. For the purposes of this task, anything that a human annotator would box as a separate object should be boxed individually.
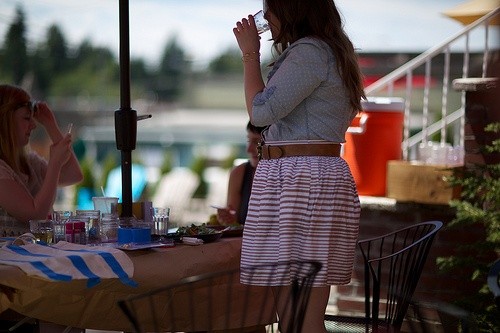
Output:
[29,209,101,249]
[92,197,120,243]
[150,207,170,237]
[412,140,465,168]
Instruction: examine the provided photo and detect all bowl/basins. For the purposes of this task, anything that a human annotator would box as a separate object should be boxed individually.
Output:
[217,208,237,226]
[389,158,452,203]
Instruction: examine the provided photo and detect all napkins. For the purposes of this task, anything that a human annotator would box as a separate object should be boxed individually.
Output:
[181,238,203,245]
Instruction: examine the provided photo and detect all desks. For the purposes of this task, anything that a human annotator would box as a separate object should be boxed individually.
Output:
[0,237,278,333]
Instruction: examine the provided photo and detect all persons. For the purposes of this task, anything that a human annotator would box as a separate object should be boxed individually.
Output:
[233,0,367,333]
[0,85,85,333]
[217,121,266,225]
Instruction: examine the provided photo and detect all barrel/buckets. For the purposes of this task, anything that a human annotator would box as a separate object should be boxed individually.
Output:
[341,96,406,197]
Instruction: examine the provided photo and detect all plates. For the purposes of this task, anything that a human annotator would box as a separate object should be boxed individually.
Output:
[208,225,242,236]
[172,228,222,244]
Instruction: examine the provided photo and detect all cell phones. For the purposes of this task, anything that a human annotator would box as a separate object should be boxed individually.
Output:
[66,123,73,136]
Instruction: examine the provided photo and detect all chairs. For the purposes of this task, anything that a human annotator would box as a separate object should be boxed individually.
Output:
[323,221,445,333]
[120,260,324,333]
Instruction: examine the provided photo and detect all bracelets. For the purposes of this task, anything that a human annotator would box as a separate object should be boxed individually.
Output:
[241,53,260,63]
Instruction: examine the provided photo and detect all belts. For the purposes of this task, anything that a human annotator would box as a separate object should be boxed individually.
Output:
[257,141,341,160]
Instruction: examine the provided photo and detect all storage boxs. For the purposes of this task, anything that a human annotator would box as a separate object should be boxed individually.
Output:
[117,226,152,246]
